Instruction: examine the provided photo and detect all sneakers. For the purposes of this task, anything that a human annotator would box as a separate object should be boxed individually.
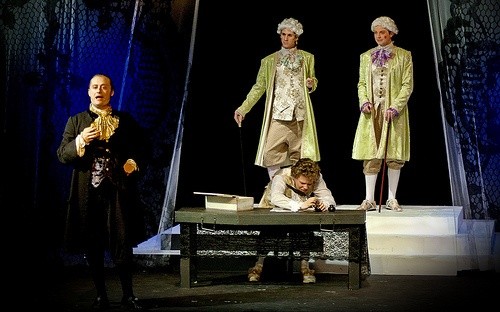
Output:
[386,200,401,212]
[303,275,316,283]
[356,200,377,211]
[248,268,260,282]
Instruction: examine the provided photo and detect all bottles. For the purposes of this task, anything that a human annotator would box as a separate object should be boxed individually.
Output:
[328,205,335,212]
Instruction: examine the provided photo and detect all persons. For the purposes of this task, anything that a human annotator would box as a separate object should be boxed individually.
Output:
[249,158,336,283]
[234,18,321,181]
[352,16,414,212]
[57,74,149,312]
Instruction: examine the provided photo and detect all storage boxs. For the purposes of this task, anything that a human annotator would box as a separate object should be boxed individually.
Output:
[194,191,254,212]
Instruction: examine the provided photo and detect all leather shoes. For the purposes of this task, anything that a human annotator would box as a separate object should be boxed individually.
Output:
[90,297,108,312]
[121,295,148,312]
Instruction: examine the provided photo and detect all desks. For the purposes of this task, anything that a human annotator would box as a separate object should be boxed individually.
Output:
[175,208,366,290]
